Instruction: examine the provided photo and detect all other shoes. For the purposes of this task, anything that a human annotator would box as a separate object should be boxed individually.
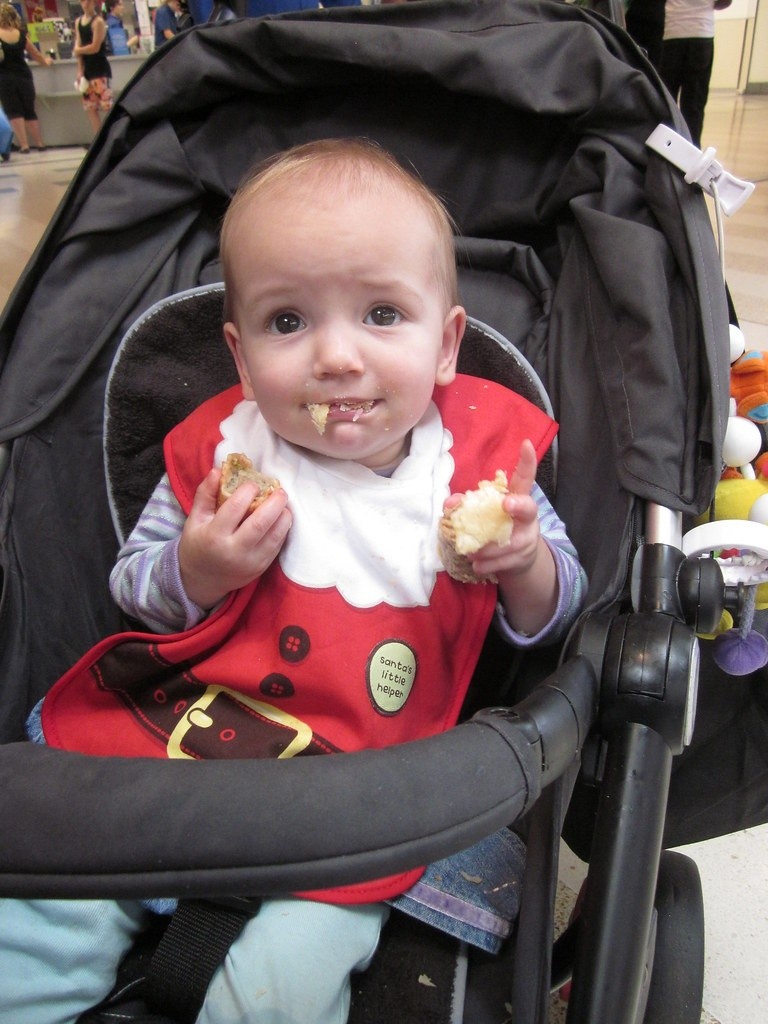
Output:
[39,145,47,151]
[19,149,30,154]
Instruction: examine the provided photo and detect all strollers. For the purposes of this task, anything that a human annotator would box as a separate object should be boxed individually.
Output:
[0,0,728,1024]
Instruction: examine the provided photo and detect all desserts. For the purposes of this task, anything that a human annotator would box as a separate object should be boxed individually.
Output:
[216,452,281,525]
[434,468,513,584]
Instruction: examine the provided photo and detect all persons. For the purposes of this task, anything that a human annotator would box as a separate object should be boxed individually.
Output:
[0,140,588,1024]
[72,0,114,135]
[154,0,182,50]
[0,3,52,153]
[207,0,236,22]
[102,0,138,55]
[657,0,732,150]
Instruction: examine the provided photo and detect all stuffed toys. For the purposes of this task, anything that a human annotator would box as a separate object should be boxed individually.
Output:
[720,350,768,480]
